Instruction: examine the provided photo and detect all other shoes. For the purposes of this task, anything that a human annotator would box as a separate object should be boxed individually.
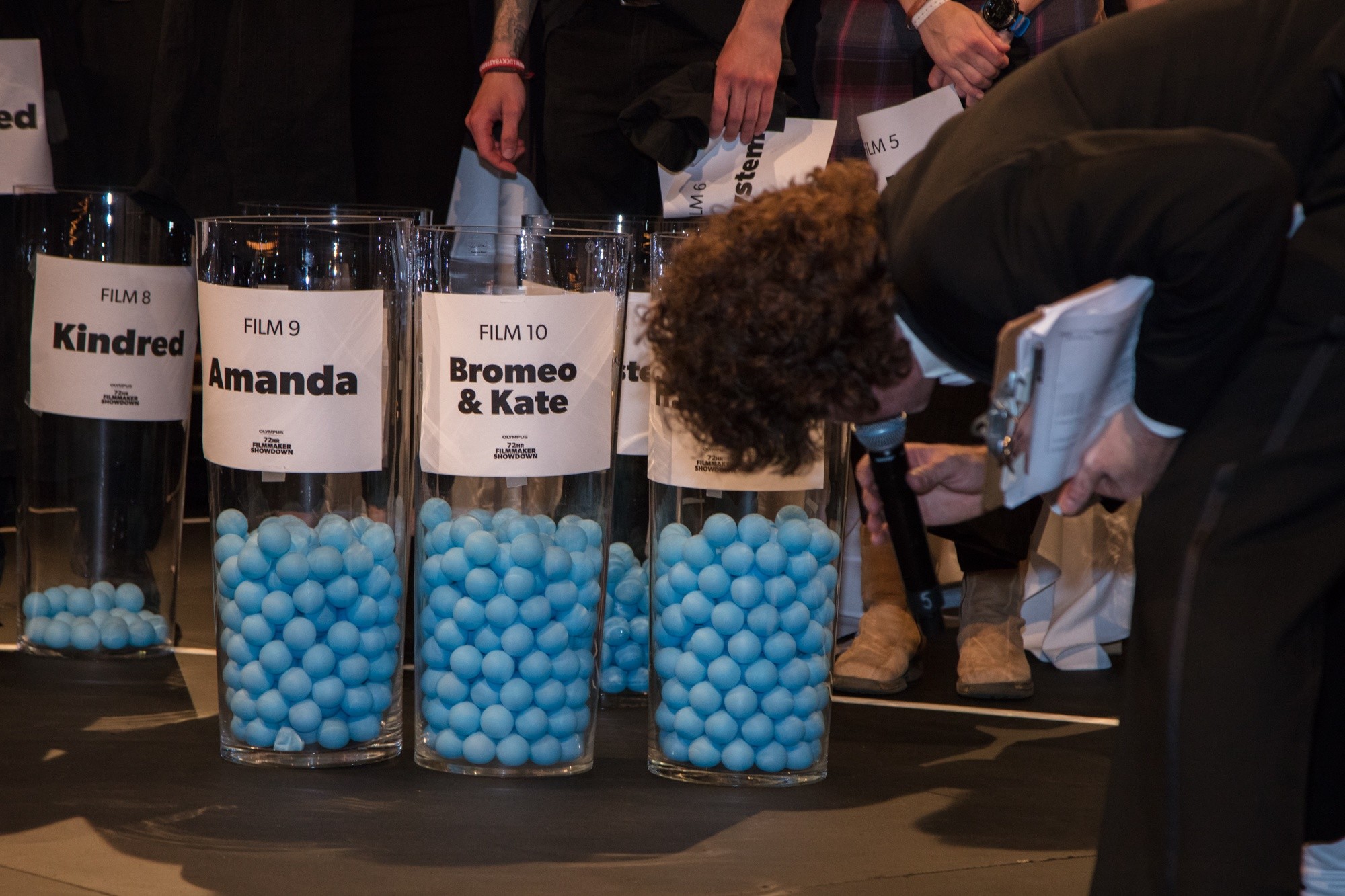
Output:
[71,522,160,613]
[1301,838,1345,896]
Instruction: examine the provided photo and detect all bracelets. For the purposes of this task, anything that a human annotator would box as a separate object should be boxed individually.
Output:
[912,0,950,30]
[478,56,535,81]
[905,0,930,24]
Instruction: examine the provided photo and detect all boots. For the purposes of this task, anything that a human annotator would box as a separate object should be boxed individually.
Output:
[958,562,1034,697]
[833,522,924,692]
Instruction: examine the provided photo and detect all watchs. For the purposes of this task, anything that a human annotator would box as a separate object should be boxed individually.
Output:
[981,0,1031,38]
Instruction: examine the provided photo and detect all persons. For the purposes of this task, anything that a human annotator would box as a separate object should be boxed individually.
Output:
[636,0,1345,896]
[463,0,1144,699]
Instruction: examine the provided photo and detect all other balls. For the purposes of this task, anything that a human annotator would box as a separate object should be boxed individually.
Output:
[649,503,843,775]
[416,497,605,770]
[600,543,652,694]
[214,507,405,752]
[22,580,170,652]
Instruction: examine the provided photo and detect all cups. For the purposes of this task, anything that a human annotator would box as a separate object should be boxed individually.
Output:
[0,185,862,792]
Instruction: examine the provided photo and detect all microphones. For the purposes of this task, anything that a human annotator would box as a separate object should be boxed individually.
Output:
[856,414,946,638]
